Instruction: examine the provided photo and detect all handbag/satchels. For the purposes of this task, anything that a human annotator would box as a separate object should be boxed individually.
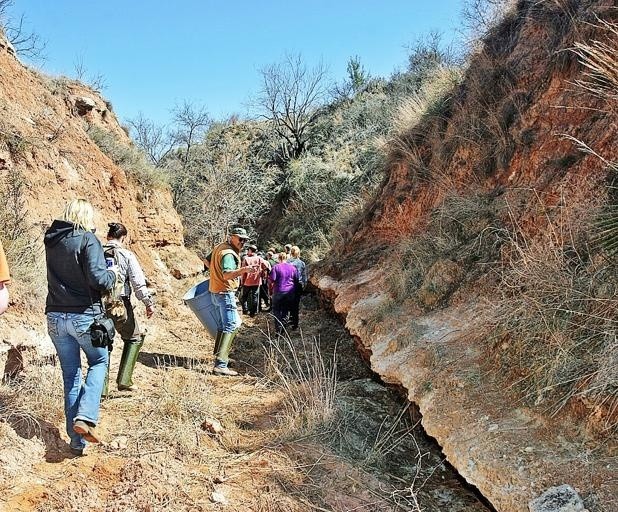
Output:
[89,317,116,348]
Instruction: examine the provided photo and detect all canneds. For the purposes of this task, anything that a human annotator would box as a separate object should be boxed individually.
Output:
[106,258,115,271]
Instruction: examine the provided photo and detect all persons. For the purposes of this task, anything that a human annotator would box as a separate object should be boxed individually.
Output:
[100,222,156,401]
[1,236,11,315]
[237,242,307,336]
[44,199,120,455]
[202,226,261,377]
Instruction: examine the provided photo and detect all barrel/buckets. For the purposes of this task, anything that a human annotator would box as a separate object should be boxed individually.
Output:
[181,279,221,342]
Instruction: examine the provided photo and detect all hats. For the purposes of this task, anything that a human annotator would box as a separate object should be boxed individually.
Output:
[244,244,293,257]
[228,227,249,243]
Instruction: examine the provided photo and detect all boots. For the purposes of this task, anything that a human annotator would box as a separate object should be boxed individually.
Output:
[211,329,240,376]
[116,335,146,391]
[99,350,111,401]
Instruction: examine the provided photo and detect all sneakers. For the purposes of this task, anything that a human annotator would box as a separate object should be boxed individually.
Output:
[69,445,83,456]
[274,317,299,338]
[239,301,271,318]
[73,420,100,443]
